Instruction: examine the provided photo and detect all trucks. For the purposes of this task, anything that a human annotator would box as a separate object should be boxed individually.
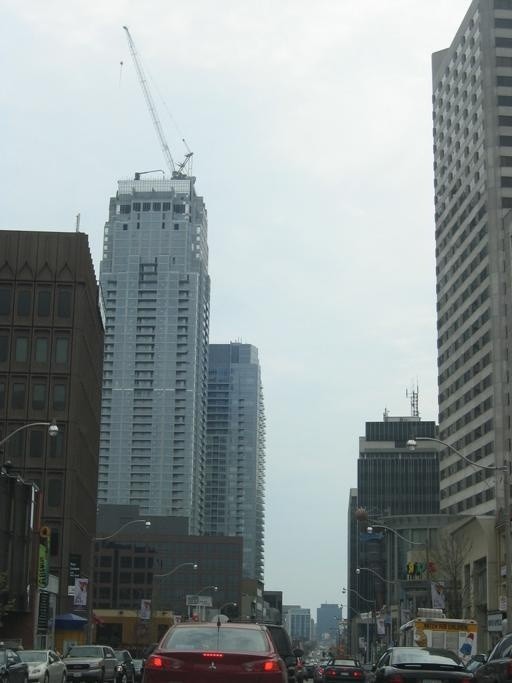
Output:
[396,606,478,665]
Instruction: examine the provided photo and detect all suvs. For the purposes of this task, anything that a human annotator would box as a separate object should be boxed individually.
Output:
[113,648,135,682]
[62,643,116,681]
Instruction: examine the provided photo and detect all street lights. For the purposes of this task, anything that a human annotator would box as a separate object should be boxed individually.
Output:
[368,525,431,581]
[0,419,58,452]
[86,517,151,644]
[355,567,402,631]
[217,601,238,616]
[341,585,376,605]
[337,603,362,622]
[191,583,218,598]
[147,561,198,642]
[406,434,512,632]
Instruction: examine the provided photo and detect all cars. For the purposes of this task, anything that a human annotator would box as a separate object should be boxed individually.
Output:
[465,651,490,671]
[143,615,289,682]
[475,632,512,682]
[368,645,477,682]
[264,621,366,682]
[0,646,29,682]
[131,657,144,678]
[15,649,67,682]
